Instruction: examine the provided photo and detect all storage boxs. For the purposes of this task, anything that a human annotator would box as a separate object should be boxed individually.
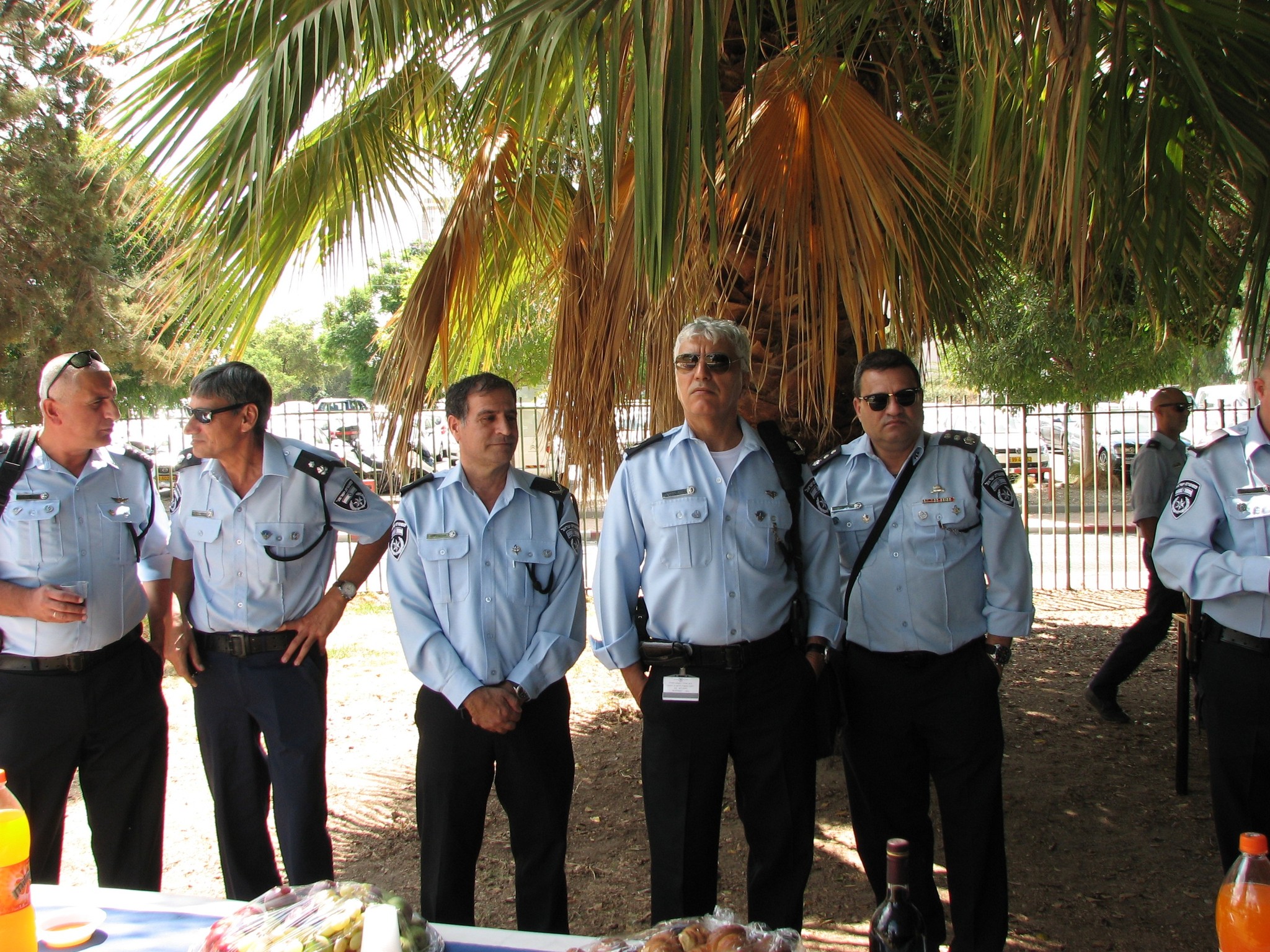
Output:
[336,425,361,441]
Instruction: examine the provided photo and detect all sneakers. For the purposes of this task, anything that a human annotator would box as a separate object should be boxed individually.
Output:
[1084,686,1133,725]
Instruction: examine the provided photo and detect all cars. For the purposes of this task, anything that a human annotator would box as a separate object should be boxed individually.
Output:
[930,404,1049,483]
[124,409,206,490]
[514,400,569,479]
[1040,402,1195,484]
[545,397,652,489]
[273,397,461,492]
[1121,383,1259,436]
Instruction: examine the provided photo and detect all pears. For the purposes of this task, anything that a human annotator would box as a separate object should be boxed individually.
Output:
[376,893,431,952]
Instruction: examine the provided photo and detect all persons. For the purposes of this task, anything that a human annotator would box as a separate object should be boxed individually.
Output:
[813,349,1035,951]
[1086,357,1270,882]
[164,361,395,903]
[383,374,587,933]
[0,353,174,894]
[590,317,840,940]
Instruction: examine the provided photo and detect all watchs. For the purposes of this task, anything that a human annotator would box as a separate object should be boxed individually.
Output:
[985,644,1012,667]
[806,643,831,665]
[509,680,530,702]
[333,579,357,601]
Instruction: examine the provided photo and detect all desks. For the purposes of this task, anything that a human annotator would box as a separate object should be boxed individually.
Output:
[29,882,604,952]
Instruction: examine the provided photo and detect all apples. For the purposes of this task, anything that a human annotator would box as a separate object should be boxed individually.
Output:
[201,880,335,952]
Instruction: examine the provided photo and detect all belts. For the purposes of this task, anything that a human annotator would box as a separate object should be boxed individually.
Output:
[690,627,793,672]
[1208,623,1270,654]
[0,622,142,671]
[893,645,996,665]
[194,631,296,657]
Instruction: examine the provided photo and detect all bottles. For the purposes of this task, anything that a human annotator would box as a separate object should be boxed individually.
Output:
[1215,832,1270,952]
[0,768,38,952]
[869,838,928,952]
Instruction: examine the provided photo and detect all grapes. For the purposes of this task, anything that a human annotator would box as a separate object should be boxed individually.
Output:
[237,883,375,952]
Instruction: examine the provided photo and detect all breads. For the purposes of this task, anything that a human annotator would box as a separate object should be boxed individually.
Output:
[567,924,792,952]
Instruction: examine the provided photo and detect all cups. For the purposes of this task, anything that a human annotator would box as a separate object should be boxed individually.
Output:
[61,581,89,619]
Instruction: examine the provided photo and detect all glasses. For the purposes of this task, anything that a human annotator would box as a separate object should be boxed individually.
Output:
[1157,402,1194,413]
[46,349,104,398]
[674,352,744,374]
[183,401,247,424]
[858,388,921,412]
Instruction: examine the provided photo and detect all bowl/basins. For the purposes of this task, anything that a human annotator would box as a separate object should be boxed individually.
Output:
[38,908,106,948]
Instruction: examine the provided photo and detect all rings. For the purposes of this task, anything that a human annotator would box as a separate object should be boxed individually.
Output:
[53,611,56,618]
